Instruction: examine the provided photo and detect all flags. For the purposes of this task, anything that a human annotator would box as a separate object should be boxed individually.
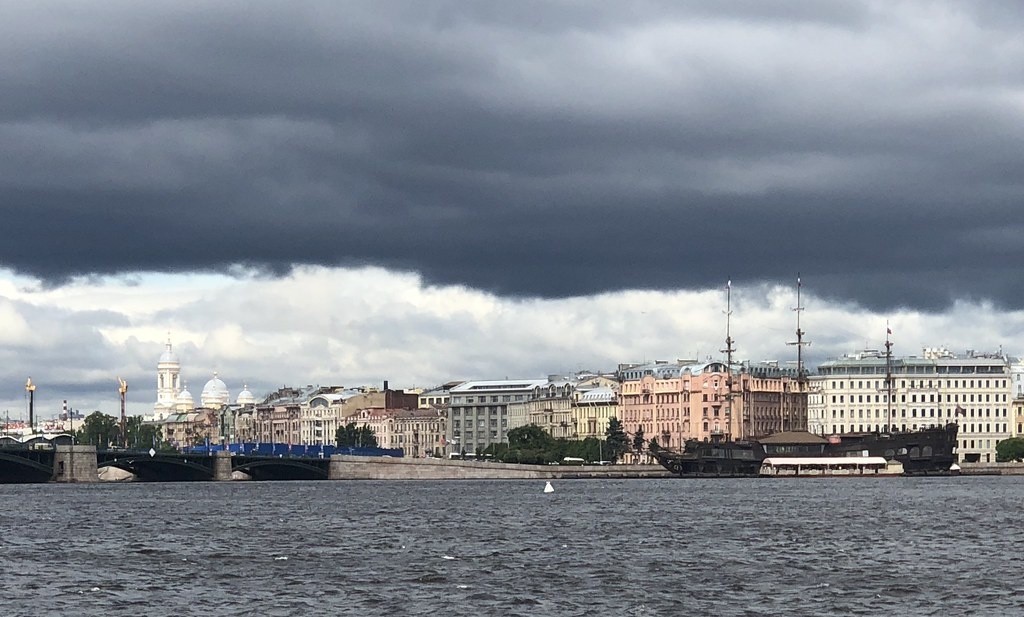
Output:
[957,405,965,414]
[887,328,891,334]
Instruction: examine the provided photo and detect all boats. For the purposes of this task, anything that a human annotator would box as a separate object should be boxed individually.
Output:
[757,457,908,477]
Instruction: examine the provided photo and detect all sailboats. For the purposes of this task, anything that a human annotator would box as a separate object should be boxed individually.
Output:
[645,273,959,477]
